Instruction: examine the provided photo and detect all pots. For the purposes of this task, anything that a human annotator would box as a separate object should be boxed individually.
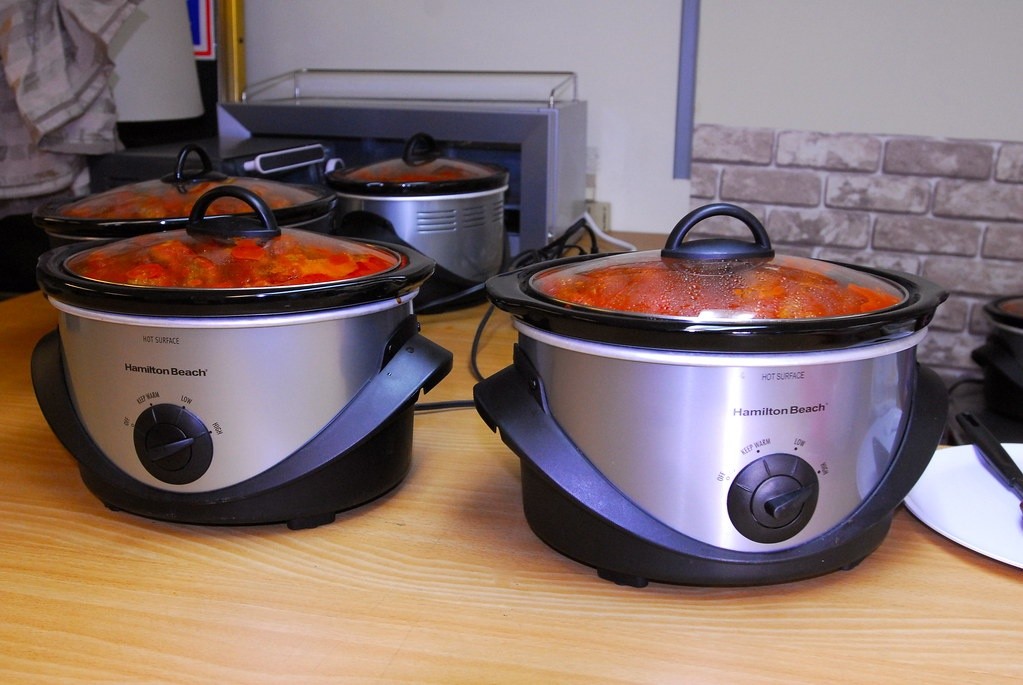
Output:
[972,294,1023,415]
[30,185,455,530]
[326,134,510,281]
[31,144,336,248]
[473,199,949,586]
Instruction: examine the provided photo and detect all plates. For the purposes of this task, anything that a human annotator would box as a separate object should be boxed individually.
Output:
[903,438,1023,570]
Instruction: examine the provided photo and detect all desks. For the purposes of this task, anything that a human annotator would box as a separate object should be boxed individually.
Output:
[0,229,1023,685]
